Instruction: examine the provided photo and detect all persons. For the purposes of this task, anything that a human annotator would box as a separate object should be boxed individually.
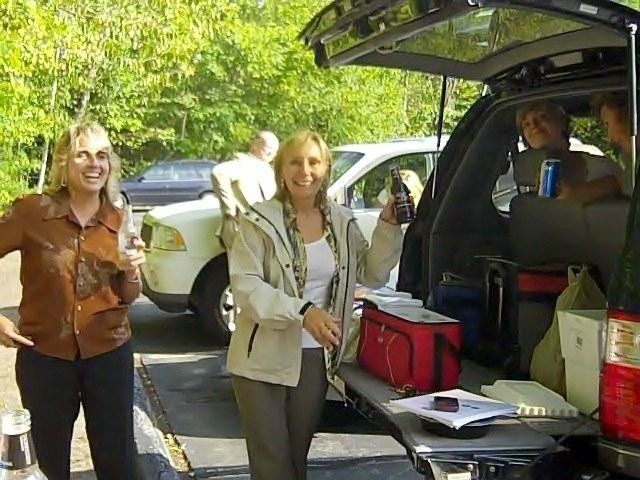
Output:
[1,121,147,479]
[211,130,281,255]
[377,169,424,236]
[515,100,622,184]
[227,130,415,480]
[556,91,635,209]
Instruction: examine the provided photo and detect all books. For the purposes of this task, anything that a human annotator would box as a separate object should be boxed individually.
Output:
[381,387,603,470]
[352,281,461,325]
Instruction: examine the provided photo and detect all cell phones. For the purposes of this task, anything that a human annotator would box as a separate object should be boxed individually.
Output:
[434,394,459,413]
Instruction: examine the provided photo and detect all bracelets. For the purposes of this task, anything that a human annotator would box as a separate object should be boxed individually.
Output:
[125,270,141,287]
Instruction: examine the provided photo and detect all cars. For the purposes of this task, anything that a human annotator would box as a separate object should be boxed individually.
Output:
[116,157,220,210]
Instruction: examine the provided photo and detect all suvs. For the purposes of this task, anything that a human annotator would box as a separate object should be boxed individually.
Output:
[291,3,639,477]
[136,131,448,343]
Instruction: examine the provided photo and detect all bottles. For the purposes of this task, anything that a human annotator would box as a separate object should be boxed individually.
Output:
[0,409,49,480]
[390,166,415,224]
[115,202,137,269]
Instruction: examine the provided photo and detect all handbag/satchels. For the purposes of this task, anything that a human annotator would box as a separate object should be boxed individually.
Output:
[354,284,462,393]
[530,265,607,393]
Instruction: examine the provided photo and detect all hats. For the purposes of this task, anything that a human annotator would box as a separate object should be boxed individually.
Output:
[377,170,425,208]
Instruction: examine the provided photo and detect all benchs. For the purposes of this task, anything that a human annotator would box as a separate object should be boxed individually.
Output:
[509,151,631,342]
